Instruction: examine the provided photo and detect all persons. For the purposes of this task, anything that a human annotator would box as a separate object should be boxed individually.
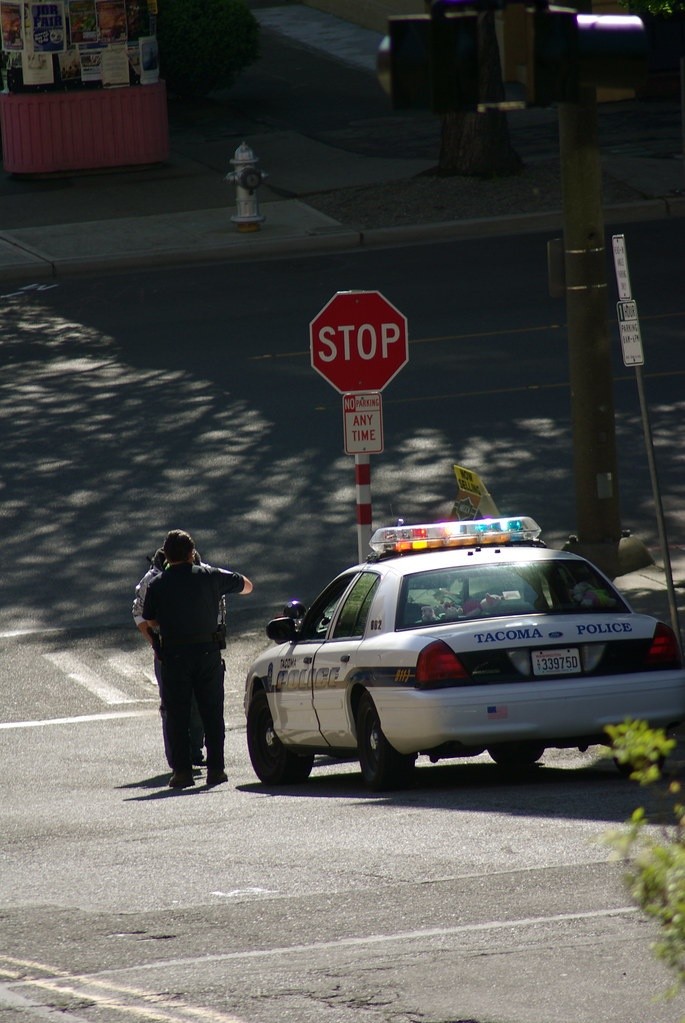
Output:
[132,530,252,787]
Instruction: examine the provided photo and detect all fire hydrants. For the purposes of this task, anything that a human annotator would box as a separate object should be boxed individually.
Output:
[220,140,268,233]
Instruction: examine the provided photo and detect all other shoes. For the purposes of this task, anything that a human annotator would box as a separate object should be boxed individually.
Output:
[206,768,227,784]
[168,771,195,788]
[193,760,207,766]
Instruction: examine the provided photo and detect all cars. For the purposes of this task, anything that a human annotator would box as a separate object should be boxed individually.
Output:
[243,515,684,789]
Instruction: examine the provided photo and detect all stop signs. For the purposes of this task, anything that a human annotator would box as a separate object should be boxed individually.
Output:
[309,290,411,393]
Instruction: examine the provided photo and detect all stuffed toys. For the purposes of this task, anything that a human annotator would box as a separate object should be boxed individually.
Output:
[422,593,501,623]
[573,581,616,606]
